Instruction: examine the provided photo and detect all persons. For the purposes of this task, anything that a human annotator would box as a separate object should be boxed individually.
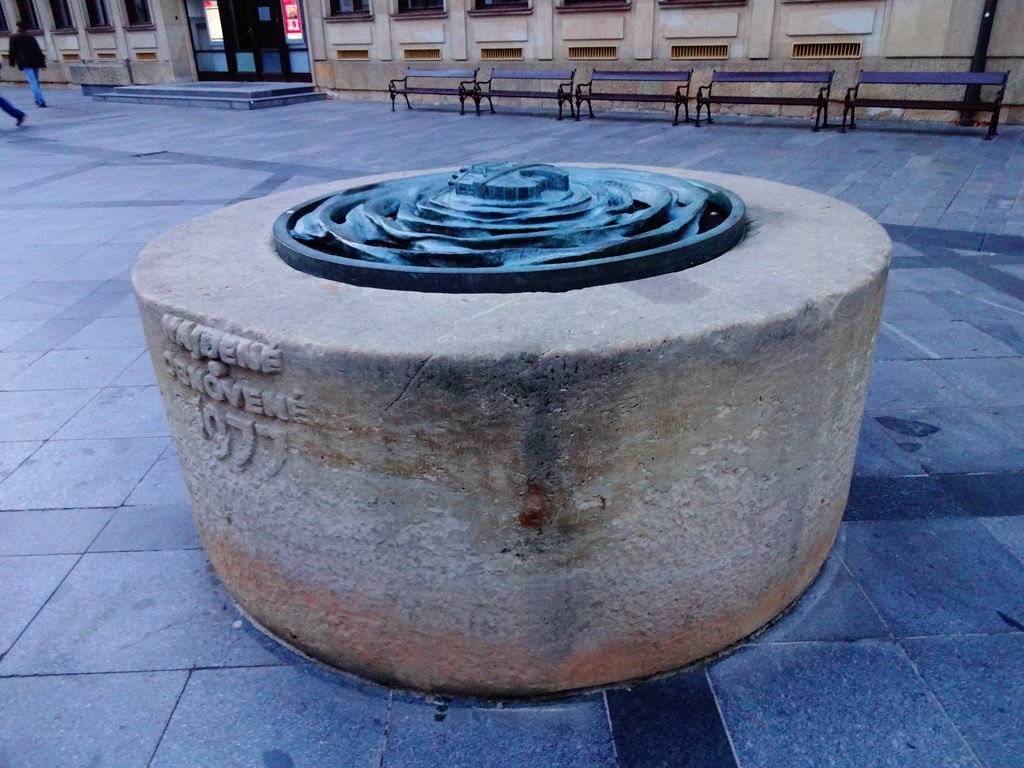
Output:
[0,62,26,126]
[9,21,47,107]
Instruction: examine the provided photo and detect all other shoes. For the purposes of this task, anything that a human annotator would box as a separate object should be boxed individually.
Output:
[39,102,46,108]
[17,114,28,126]
[36,100,40,105]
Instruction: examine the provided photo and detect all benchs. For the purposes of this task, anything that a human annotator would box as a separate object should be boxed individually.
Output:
[473,67,576,121]
[695,70,834,133]
[389,67,480,116]
[576,69,694,126]
[840,69,1011,141]
